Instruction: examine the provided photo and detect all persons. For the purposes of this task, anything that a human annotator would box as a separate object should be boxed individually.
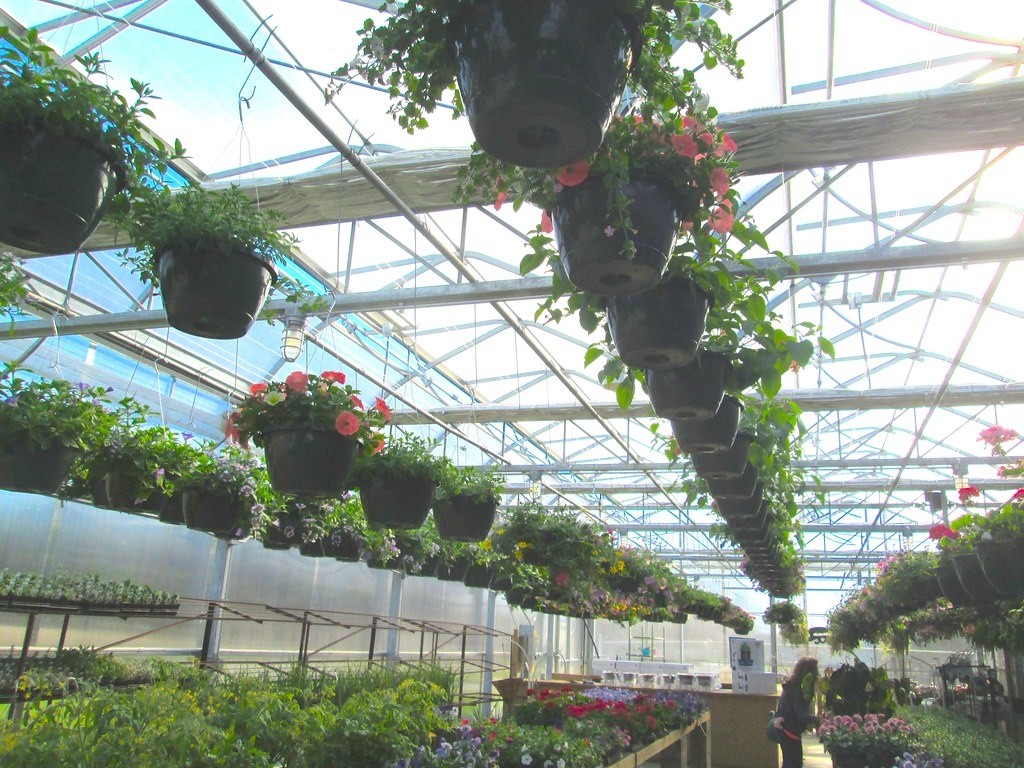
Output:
[773,656,823,768]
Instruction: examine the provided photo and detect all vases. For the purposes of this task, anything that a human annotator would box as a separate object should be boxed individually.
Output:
[950,554,997,601]
[4,411,753,635]
[936,567,970,607]
[829,752,867,768]
[456,2,788,595]
[973,540,1023,598]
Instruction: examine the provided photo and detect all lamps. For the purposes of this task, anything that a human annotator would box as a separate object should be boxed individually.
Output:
[282,308,310,360]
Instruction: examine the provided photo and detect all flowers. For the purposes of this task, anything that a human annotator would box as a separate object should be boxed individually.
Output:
[323,1,745,138]
[457,102,740,248]
[0,362,257,512]
[226,366,755,768]
[669,437,809,599]
[819,425,1024,768]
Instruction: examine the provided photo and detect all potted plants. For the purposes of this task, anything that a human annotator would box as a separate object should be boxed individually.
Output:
[0,25,325,341]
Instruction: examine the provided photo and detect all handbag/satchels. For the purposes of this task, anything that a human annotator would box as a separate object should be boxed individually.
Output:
[766,718,784,744]
